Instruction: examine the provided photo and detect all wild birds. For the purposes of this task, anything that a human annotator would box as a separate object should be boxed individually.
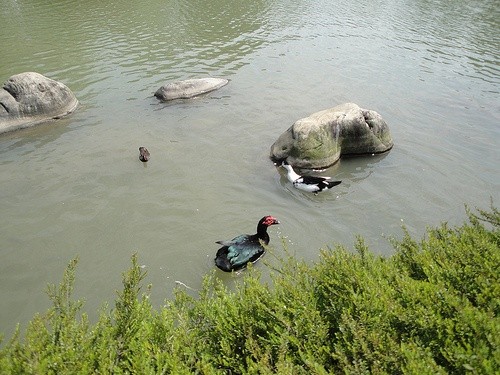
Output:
[138,146,151,162]
[273,157,343,196]
[214,215,280,272]
